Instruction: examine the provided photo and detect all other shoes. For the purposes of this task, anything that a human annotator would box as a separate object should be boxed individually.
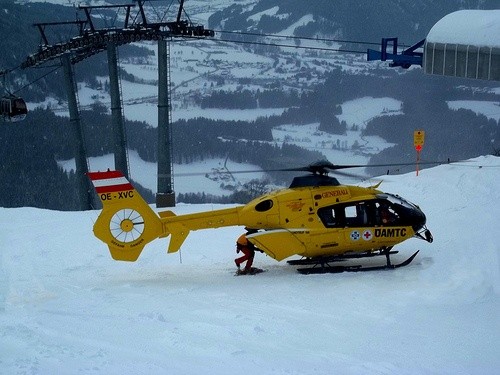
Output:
[245,268,252,271]
[235,259,240,267]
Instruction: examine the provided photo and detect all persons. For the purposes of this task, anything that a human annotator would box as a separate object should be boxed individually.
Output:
[235,231,264,271]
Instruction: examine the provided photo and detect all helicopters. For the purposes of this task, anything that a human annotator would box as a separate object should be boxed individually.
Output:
[84,161,479,275]
